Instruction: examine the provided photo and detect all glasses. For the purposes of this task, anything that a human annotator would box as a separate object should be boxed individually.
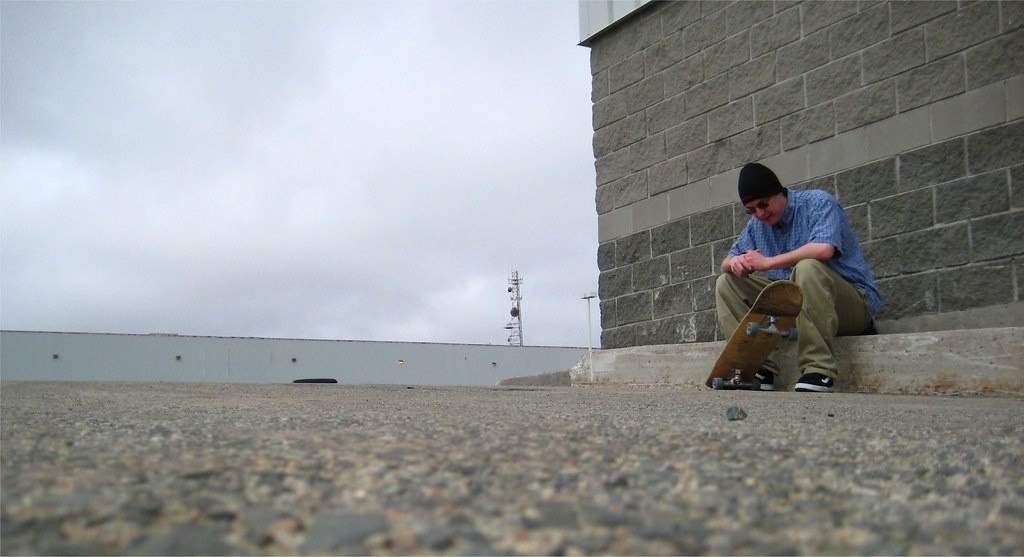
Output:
[745,197,772,215]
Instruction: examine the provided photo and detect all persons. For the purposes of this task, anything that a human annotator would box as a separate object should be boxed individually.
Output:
[715,162,884,392]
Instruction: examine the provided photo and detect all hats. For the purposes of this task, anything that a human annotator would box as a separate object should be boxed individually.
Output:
[739,162,784,206]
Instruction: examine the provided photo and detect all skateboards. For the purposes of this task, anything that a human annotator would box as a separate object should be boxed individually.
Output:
[704,280,804,390]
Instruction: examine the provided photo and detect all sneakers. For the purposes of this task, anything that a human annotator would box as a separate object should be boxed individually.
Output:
[795,372,843,393]
[755,368,774,390]
